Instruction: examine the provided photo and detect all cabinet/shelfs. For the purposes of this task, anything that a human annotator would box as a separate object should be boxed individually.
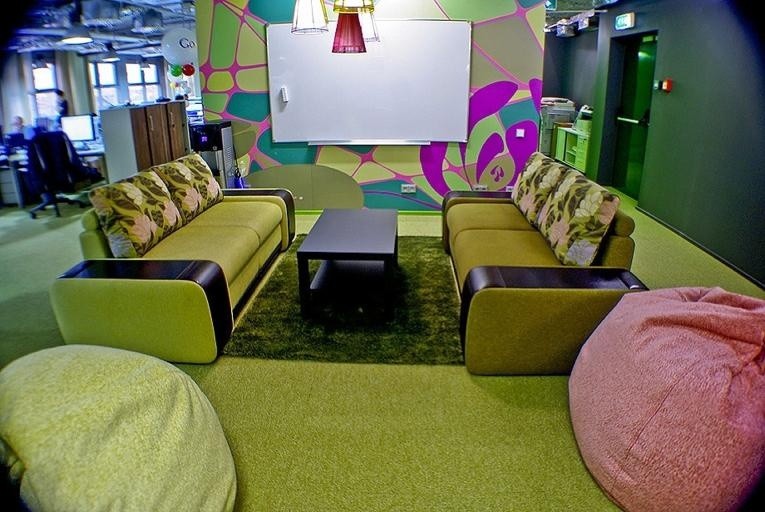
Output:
[140,101,173,166]
[554,124,591,178]
[163,98,191,162]
[98,106,153,185]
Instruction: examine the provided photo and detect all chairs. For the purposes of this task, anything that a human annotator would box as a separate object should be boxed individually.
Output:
[25,128,100,222]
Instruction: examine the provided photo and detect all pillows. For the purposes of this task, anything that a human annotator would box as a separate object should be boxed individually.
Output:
[511,150,570,231]
[86,168,184,259]
[535,168,622,267]
[152,150,225,227]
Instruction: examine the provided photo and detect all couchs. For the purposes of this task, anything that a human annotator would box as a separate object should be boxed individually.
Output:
[440,149,651,377]
[47,151,295,364]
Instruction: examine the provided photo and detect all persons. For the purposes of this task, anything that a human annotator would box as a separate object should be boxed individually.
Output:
[10,115,25,133]
[184,94,188,100]
[54,89,69,125]
[175,95,184,100]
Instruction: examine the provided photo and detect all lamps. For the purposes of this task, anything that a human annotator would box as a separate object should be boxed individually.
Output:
[139,55,150,70]
[74,1,126,28]
[60,10,96,45]
[130,2,167,34]
[289,1,381,54]
[100,38,120,63]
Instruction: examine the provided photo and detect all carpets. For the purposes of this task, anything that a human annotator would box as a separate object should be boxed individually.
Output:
[222,232,467,368]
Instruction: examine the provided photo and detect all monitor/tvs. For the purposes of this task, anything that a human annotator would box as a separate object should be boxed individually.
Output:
[60,114,96,150]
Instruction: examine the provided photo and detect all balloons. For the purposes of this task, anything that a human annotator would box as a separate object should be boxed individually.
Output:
[159,28,199,94]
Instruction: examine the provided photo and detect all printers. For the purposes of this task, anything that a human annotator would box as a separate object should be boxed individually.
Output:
[538,97,576,157]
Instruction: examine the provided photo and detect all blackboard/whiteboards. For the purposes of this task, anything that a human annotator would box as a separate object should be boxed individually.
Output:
[263,18,474,146]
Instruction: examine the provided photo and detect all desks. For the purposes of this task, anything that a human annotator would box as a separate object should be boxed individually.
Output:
[0,149,104,211]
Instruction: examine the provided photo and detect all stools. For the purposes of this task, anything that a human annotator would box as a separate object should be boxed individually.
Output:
[562,279,765,511]
[0,344,242,511]
